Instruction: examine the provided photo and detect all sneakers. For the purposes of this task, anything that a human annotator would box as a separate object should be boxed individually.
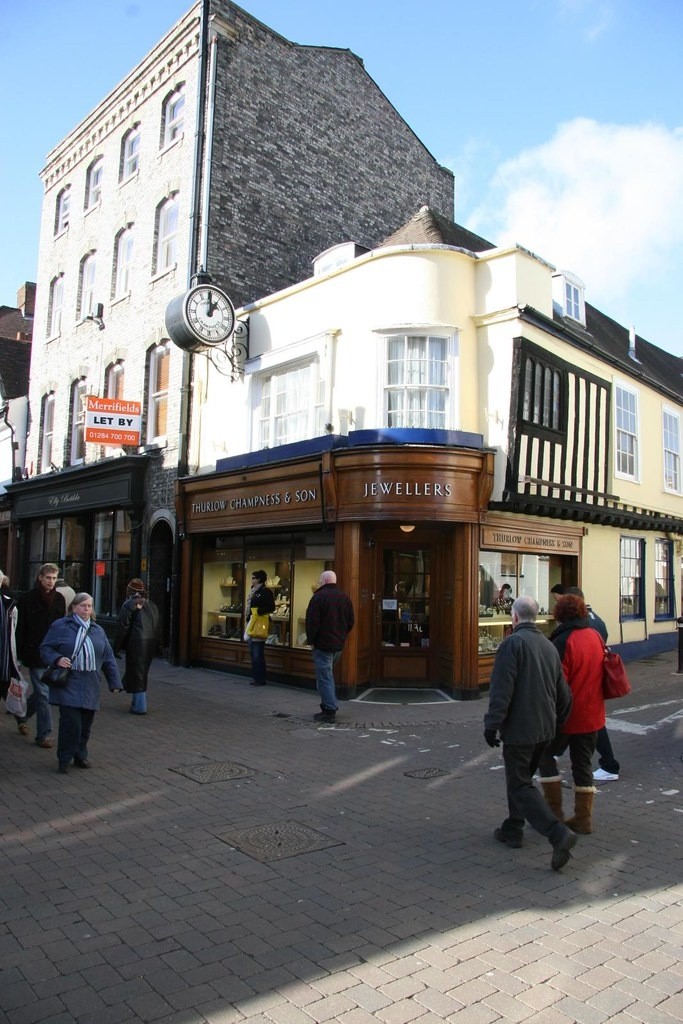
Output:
[592,768,619,781]
[553,756,559,766]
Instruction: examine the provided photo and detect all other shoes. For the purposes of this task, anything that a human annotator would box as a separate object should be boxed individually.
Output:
[549,825,578,869]
[18,723,28,735]
[495,819,523,848]
[36,738,53,747]
[254,675,266,686]
[74,759,91,768]
[314,704,335,723]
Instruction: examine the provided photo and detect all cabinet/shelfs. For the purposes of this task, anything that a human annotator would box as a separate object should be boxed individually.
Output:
[202,560,334,649]
[479,562,573,657]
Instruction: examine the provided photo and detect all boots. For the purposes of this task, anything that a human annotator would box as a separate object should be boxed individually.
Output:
[563,786,596,834]
[541,775,563,822]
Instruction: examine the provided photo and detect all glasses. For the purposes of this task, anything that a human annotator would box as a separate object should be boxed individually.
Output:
[252,577,257,579]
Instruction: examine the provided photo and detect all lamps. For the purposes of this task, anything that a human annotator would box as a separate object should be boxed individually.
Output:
[400,521,416,533]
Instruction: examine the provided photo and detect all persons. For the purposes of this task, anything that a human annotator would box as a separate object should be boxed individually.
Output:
[306,571,355,723]
[538,596,609,834]
[495,583,515,612]
[40,593,123,774]
[552,584,619,780]
[245,570,276,686]
[483,595,578,869]
[0,563,78,748]
[117,578,160,716]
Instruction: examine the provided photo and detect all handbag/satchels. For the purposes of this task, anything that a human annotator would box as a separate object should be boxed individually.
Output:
[41,666,70,687]
[5,677,29,717]
[604,654,631,700]
[244,621,267,642]
[247,608,269,639]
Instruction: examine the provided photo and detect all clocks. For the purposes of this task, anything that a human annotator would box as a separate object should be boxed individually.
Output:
[165,265,251,383]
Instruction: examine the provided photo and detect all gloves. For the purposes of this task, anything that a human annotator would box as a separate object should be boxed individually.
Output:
[484,730,500,748]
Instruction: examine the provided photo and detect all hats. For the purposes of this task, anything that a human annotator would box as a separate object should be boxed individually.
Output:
[567,587,583,596]
[551,584,565,595]
[128,578,144,591]
[253,570,267,581]
